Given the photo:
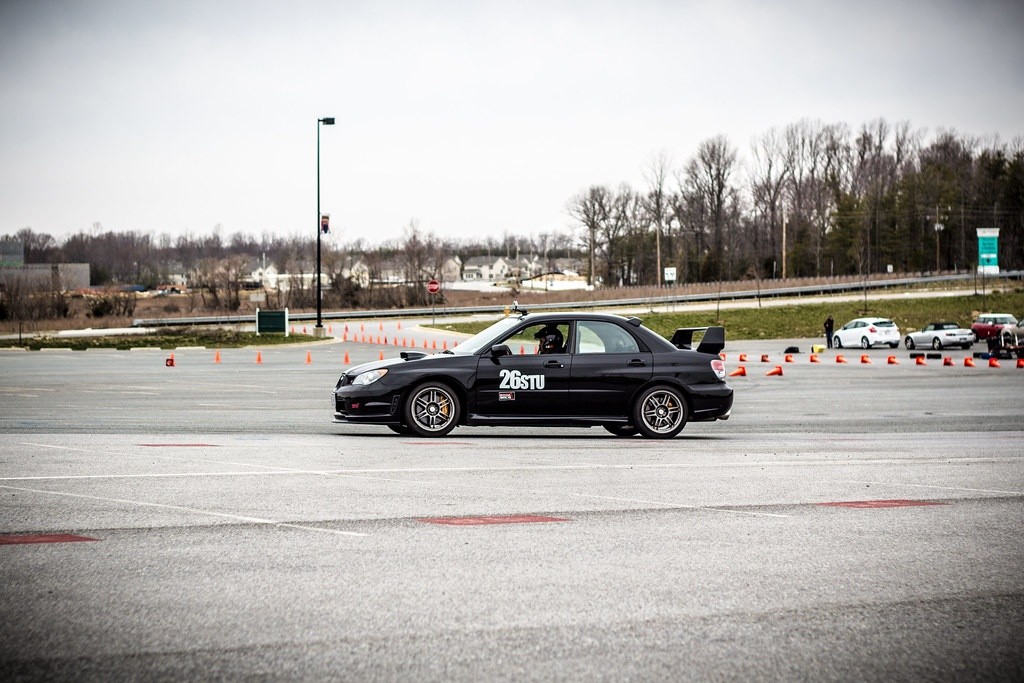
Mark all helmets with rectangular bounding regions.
[534,327,563,351]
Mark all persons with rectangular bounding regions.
[824,315,834,349]
[534,327,564,354]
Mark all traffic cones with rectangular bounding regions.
[944,357,954,365]
[534,344,539,355]
[785,355,794,363]
[520,346,525,355]
[888,356,899,364]
[767,366,782,376]
[256,351,263,364]
[344,351,350,365]
[729,366,747,377]
[762,355,770,362]
[740,354,748,362]
[170,353,175,366]
[916,356,927,366]
[861,354,872,363]
[964,357,976,367]
[305,350,312,364]
[291,321,458,351]
[214,350,222,363]
[988,358,1001,368]
[811,354,820,363]
[835,354,847,364]
[379,351,383,360]
[1016,357,1024,368]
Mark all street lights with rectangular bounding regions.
[312,117,335,337]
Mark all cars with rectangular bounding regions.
[831,316,900,350]
[1001,320,1024,353]
[332,306,733,439]
[971,313,1019,343]
[904,322,976,350]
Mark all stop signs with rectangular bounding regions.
[427,280,439,293]
